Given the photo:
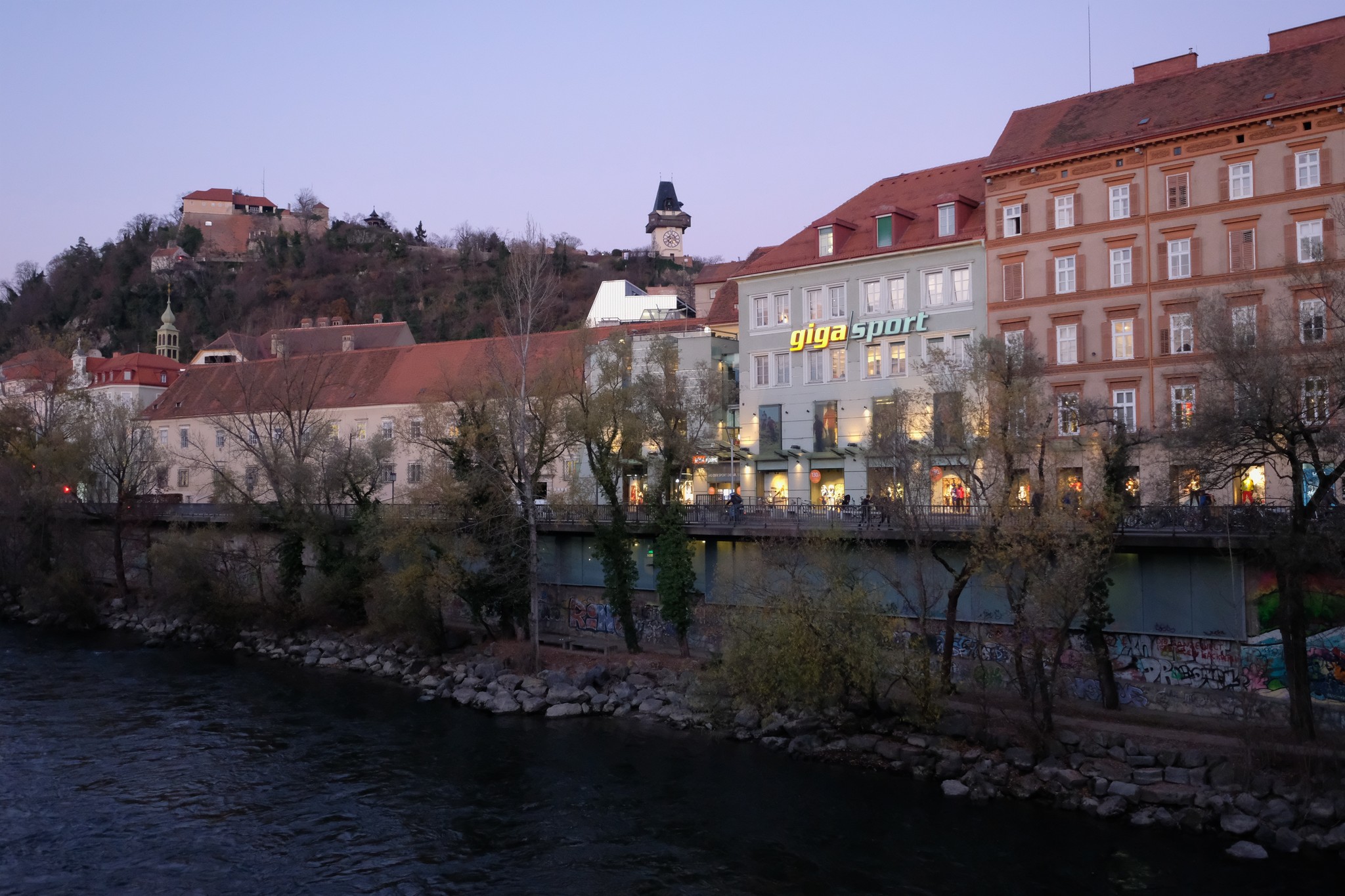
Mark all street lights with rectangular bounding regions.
[247,477,253,506]
[389,473,397,506]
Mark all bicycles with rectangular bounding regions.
[720,505,747,524]
[827,495,868,520]
[1122,498,1333,536]
[797,498,816,517]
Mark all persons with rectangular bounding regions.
[1060,491,1071,510]
[1240,472,1255,504]
[631,485,635,502]
[1018,486,1027,506]
[1068,476,1083,491]
[724,493,740,520]
[636,490,644,505]
[813,401,836,452]
[760,410,778,439]
[687,482,692,493]
[766,493,773,505]
[372,497,382,504]
[895,482,903,504]
[1197,490,1213,529]
[937,394,955,446]
[774,485,784,498]
[820,485,836,511]
[1189,476,1200,507]
[1030,488,1041,519]
[950,484,965,514]
[857,492,894,530]
[1317,487,1345,507]
